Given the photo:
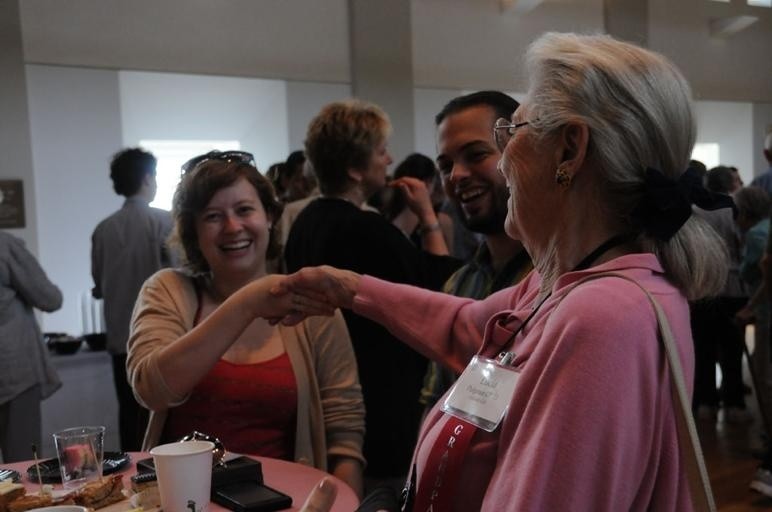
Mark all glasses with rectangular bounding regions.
[177,429,230,470]
[180,150,258,181]
[492,116,546,154]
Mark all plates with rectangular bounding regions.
[23,490,66,501]
[95,499,161,512]
[27,452,132,482]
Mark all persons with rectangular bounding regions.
[87,147,184,452]
[260,30,696,512]
[279,101,454,511]
[414,90,533,432]
[124,149,367,501]
[298,476,339,511]
[1,228,67,464]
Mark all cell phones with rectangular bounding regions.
[216,482,293,512]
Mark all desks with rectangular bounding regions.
[0,447,359,512]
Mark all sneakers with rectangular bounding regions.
[748,466,772,499]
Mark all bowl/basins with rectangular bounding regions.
[44,332,106,355]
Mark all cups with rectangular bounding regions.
[149,440,215,511]
[24,505,89,512]
[52,425,106,491]
